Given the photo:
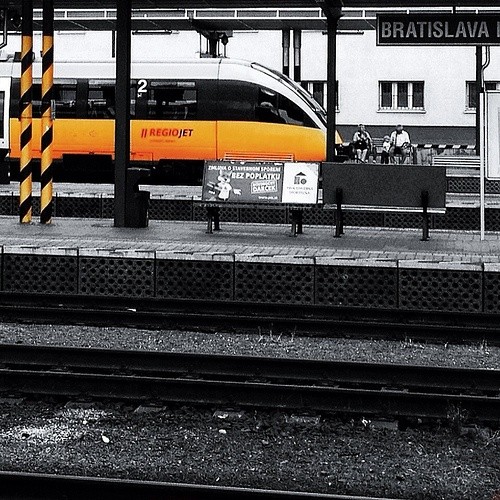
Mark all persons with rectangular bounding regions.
[353,124,373,164]
[389,125,411,164]
[380,134,391,164]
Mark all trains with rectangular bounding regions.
[0,58,344,162]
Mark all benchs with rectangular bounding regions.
[360,138,417,164]
[432,157,485,175]
[201,153,319,238]
[321,162,448,242]
